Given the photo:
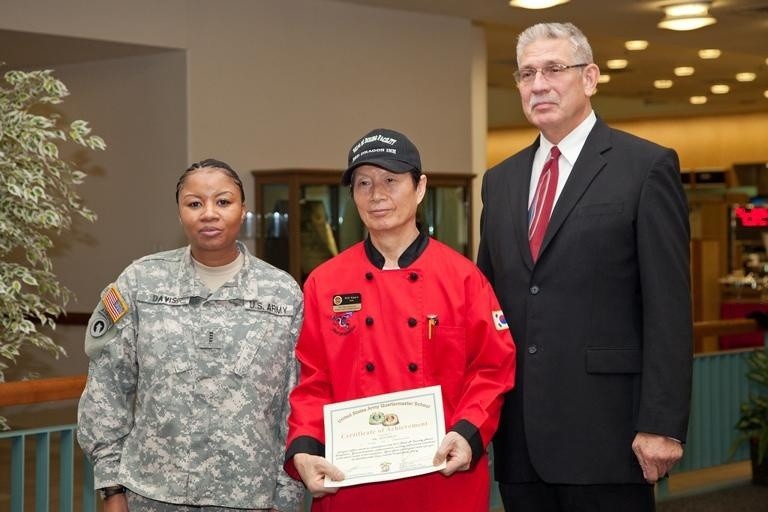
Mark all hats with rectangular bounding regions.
[341,128,421,185]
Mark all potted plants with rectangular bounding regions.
[723,347,768,493]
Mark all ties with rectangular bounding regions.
[527,147,561,264]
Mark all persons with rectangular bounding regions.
[475,22,693,512]
[77,160,304,512]
[282,130,518,512]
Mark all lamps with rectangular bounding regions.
[653,2,718,34]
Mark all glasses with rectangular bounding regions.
[512,63,587,85]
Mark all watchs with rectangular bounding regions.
[98,486,126,501]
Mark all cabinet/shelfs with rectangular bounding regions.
[246,162,478,308]
[678,157,768,358]
